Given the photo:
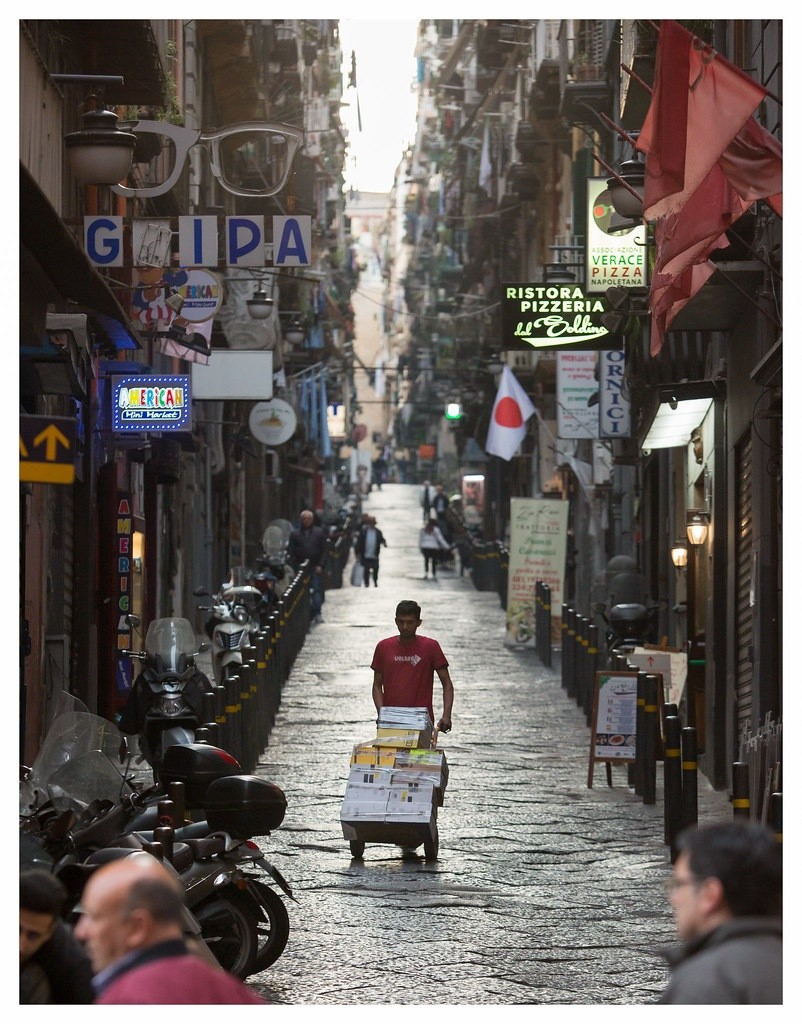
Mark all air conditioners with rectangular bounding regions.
[266,449,279,477]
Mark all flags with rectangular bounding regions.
[485,366,537,461]
[638,19,783,354]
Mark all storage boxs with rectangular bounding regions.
[162,744,243,807]
[339,706,449,823]
[204,775,287,833]
[611,603,648,637]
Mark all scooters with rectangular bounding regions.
[594,596,669,669]
[193,520,299,685]
[19,689,302,981]
[119,613,215,785]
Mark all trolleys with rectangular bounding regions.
[350,727,441,860]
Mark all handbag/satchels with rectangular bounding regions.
[351,561,366,586]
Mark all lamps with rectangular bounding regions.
[687,509,712,545]
[671,537,697,574]
[138,330,211,357]
[194,418,257,458]
[48,72,138,187]
[486,354,507,375]
[543,261,585,282]
[221,278,273,318]
[278,311,305,344]
[599,309,650,333]
[606,285,649,307]
[111,282,185,315]
[607,147,645,222]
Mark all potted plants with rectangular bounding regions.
[575,52,602,80]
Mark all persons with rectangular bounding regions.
[450,535,473,577]
[287,510,329,623]
[20,868,98,1005]
[354,465,371,495]
[432,484,449,520]
[419,479,437,523]
[351,514,387,587]
[370,599,455,734]
[371,457,386,491]
[75,847,273,1005]
[649,820,784,1006]
[420,519,451,580]
